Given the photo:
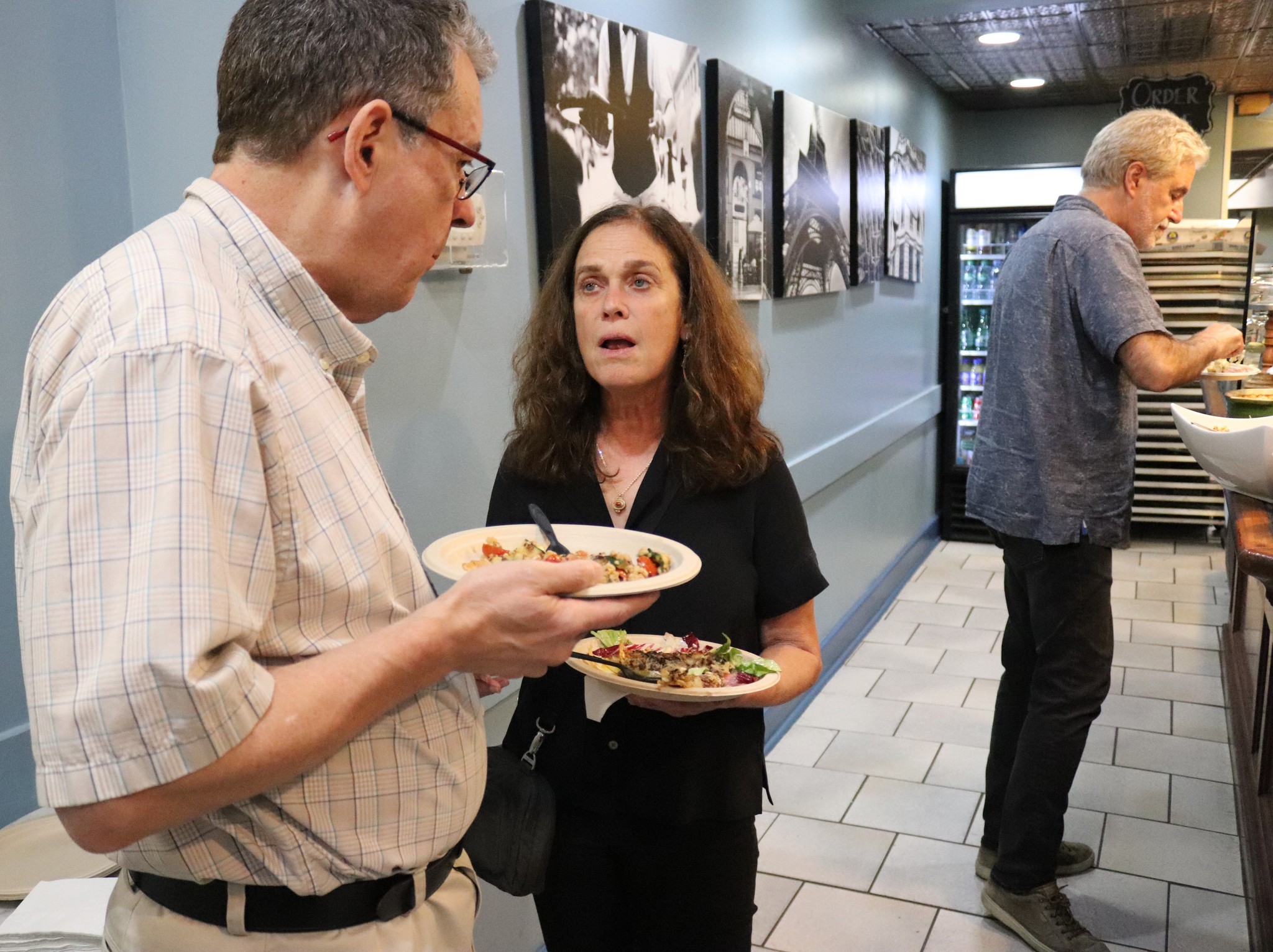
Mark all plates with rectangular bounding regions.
[1225,388,1273,405]
[1199,361,1262,381]
[564,634,780,702]
[421,523,702,599]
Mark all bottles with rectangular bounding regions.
[959,358,986,386]
[960,429,976,464]
[963,261,1003,300]
[965,222,1026,255]
[961,308,989,350]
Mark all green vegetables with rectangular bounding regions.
[605,548,664,573]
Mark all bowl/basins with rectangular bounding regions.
[1170,403,1273,503]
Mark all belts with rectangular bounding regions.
[122,834,469,934]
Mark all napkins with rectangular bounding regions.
[0,877,120,952]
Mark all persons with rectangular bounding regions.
[966,107,1247,952]
[10,1,662,952]
[485,204,830,952]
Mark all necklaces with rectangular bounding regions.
[595,443,653,514]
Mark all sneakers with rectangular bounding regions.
[980,877,1111,952]
[975,839,1095,880]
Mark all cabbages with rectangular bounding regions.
[590,628,783,677]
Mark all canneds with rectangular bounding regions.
[961,396,983,420]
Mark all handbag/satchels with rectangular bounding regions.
[460,738,555,897]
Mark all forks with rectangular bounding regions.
[570,652,662,683]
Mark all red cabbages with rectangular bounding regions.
[592,631,758,684]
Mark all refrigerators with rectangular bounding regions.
[934,161,1084,544]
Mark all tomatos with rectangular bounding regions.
[481,543,660,578]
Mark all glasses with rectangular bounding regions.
[328,105,496,200]
